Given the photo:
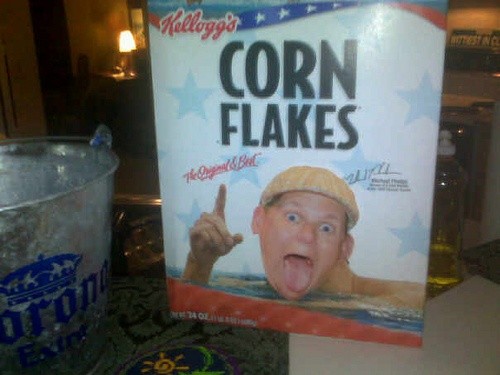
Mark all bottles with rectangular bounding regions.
[426,129,465,298]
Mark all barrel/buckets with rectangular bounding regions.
[0,124,120,375]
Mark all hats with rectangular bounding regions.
[259,165,359,230]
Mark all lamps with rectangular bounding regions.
[114,29,137,78]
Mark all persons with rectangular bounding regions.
[180,165,425,319]
